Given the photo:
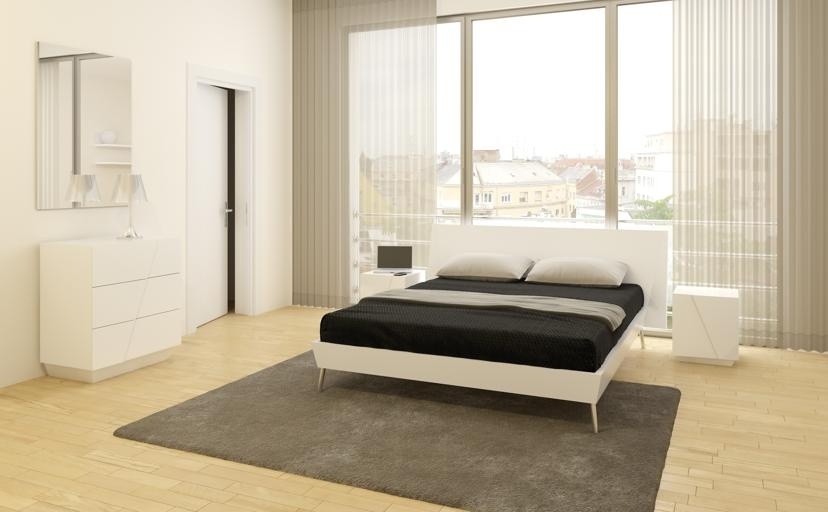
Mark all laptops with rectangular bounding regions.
[373,245,412,273]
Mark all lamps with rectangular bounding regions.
[110,174,149,239]
[69,174,104,208]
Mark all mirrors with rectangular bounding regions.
[37,39,133,210]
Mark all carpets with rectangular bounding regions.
[113,349,681,512]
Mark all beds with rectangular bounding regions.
[310,223,668,434]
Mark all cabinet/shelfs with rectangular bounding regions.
[39,233,182,383]
[94,145,133,165]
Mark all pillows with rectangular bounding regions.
[524,256,630,289]
[435,253,535,283]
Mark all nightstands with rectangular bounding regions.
[671,285,739,367]
[359,269,425,301]
